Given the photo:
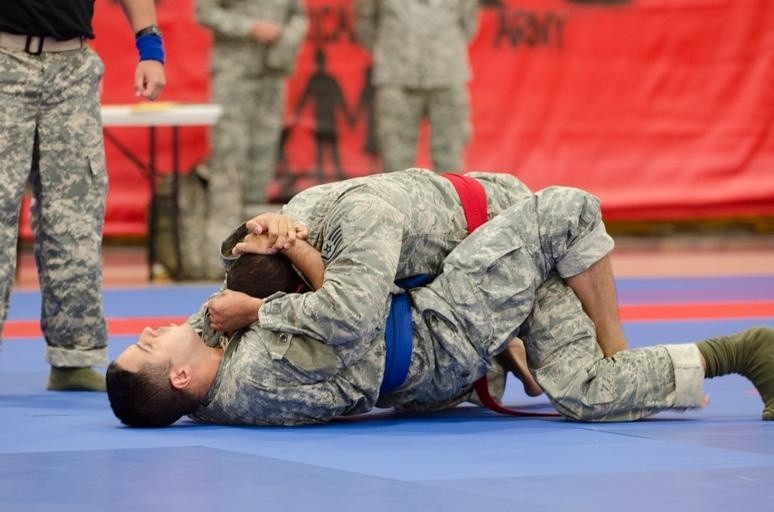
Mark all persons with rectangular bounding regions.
[206,167,774,422]
[353,0,481,174]
[0,0,167,392]
[195,0,312,283]
[106,185,632,429]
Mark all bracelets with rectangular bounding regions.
[135,35,164,64]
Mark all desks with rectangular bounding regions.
[102,102,224,282]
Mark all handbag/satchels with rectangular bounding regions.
[153,164,244,282]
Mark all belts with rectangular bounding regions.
[0,32,87,55]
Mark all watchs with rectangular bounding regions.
[135,24,162,38]
